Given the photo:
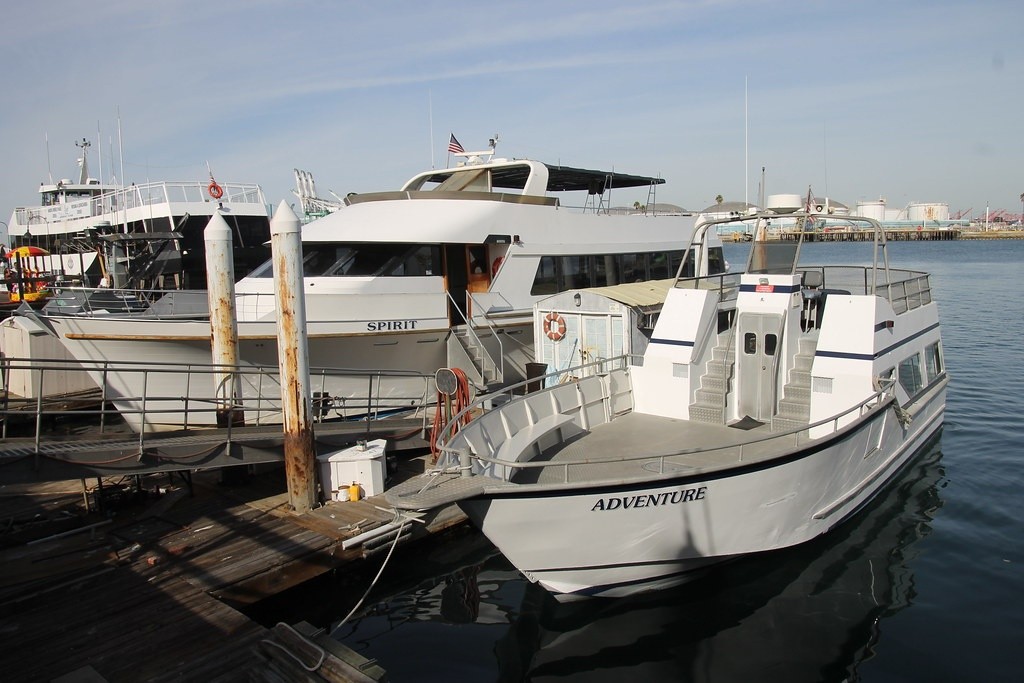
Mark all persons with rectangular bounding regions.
[0,244,18,302]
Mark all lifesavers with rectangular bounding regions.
[824,227,829,233]
[208,184,223,198]
[543,313,566,340]
[492,256,504,276]
[917,226,922,230]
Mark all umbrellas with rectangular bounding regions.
[5,246,50,258]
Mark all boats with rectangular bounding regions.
[344,164,952,602]
[0,135,732,434]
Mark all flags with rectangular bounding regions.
[449,134,468,157]
